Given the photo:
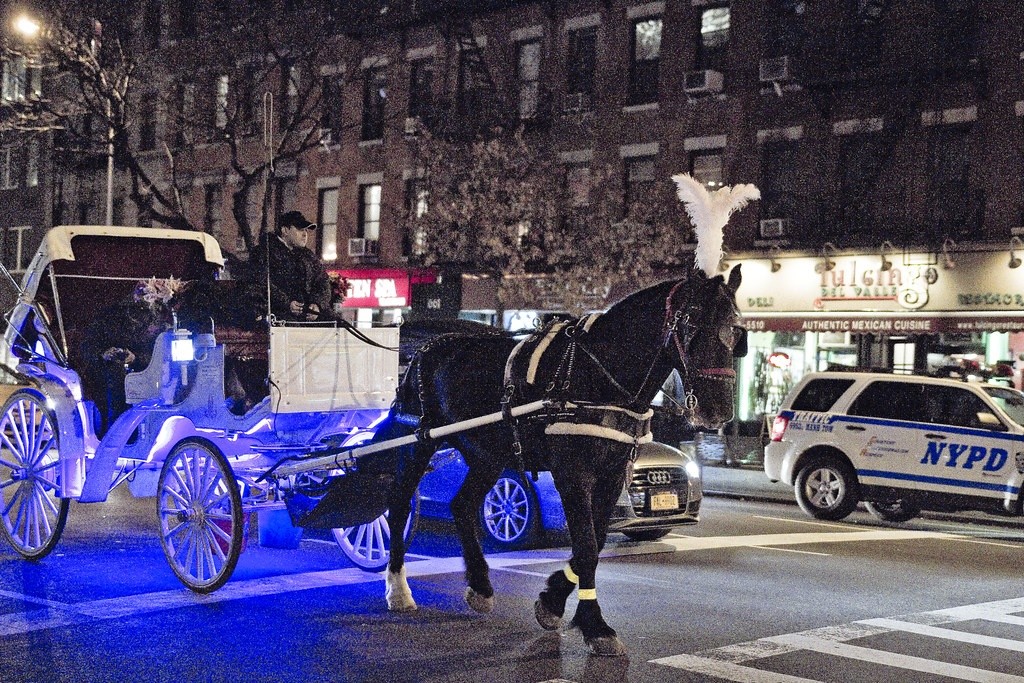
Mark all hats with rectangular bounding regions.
[278,211,316,231]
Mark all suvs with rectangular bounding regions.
[764,364,1024,523]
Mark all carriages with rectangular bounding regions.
[0,225,748,655]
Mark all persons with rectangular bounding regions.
[649,368,685,449]
[74,291,153,421]
[237,211,333,402]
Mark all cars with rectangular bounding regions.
[0,334,58,448]
[419,441,703,550]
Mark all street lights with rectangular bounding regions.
[16,19,113,226]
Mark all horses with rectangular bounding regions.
[384,263,742,656]
